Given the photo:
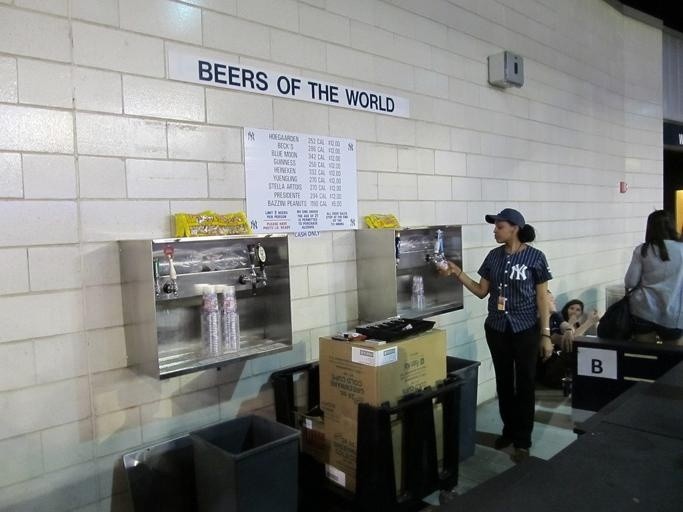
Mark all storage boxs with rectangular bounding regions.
[318,327,447,493]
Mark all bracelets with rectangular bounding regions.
[541,326,552,331]
[541,332,554,339]
[458,269,463,278]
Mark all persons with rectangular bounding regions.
[597,211,683,339]
[437,208,555,459]
[537,287,606,385]
[560,299,584,329]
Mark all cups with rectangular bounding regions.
[433,253,449,271]
[201,286,241,357]
[410,275,425,311]
[560,377,573,397]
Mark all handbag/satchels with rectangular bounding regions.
[597,296,628,341]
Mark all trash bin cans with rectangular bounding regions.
[447,356,481,456]
[189,413,302,512]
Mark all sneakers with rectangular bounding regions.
[492,430,529,462]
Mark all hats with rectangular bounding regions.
[485,209,525,230]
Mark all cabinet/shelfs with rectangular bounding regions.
[354,224,464,324]
[118,229,295,379]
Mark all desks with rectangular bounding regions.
[428,361,683,512]
